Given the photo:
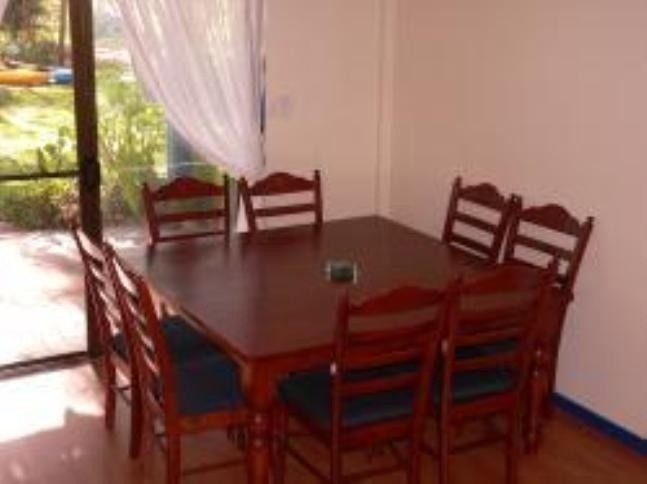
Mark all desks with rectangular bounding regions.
[113,212,547,483]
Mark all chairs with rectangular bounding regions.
[495,193,593,424]
[99,234,245,484]
[235,166,328,239]
[139,174,241,243]
[434,173,518,269]
[70,226,219,461]
[273,277,461,482]
[418,257,560,482]
